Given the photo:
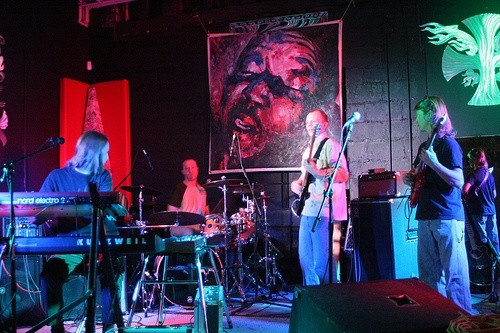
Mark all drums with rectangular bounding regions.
[199,213,236,248]
[229,211,258,245]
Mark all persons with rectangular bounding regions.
[168,158,210,230]
[36,131,113,326]
[403,95,472,312]
[463,147,500,257]
[290,109,349,286]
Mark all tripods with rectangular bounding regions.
[216,182,289,305]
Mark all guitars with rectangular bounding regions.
[294,122,322,217]
[409,115,447,209]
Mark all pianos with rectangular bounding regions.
[0,189,155,333]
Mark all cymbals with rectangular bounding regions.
[228,182,265,194]
[201,178,246,189]
[120,184,165,194]
[148,209,207,227]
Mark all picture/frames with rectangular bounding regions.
[208,19,348,174]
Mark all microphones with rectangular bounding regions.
[48,136,64,144]
[342,111,362,128]
[231,133,236,157]
[142,146,156,170]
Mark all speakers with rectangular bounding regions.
[287,277,473,333]
[0,254,44,326]
[351,197,421,284]
